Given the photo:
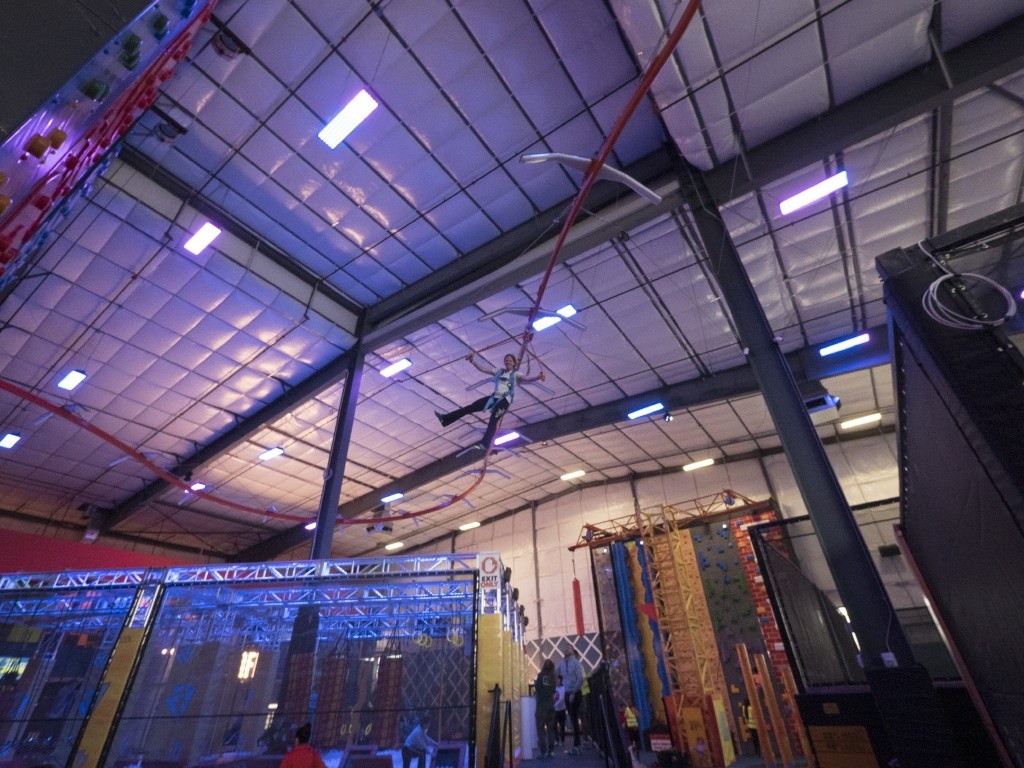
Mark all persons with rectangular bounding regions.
[554,675,567,746]
[559,643,583,756]
[535,659,557,758]
[434,353,545,450]
[403,716,438,768]
[280,721,327,768]
[624,702,640,745]
[618,702,627,723]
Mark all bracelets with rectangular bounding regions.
[571,692,575,695]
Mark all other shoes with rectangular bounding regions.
[560,741,565,748]
[434,410,444,425]
[474,444,487,451]
[564,746,579,755]
[548,750,555,756]
[555,739,559,745]
[535,754,546,759]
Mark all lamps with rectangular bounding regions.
[664,411,674,422]
[58,334,105,391]
[184,188,230,256]
[318,31,396,151]
